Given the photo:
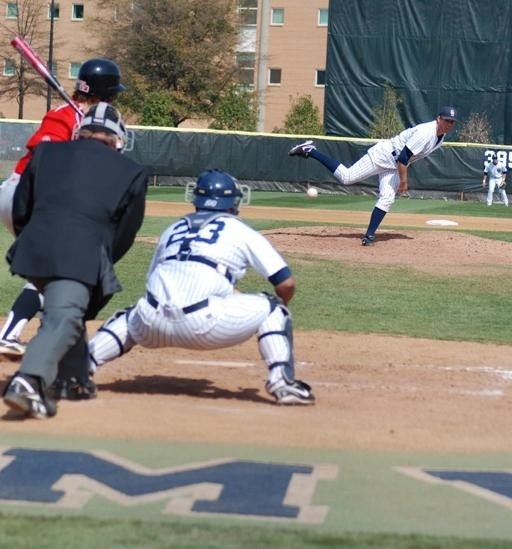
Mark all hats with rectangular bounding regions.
[439,106,457,123]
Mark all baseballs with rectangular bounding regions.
[308,188,318,198]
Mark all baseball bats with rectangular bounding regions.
[11,35,84,119]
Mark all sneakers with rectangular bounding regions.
[362,234,375,246]
[287,140,315,159]
[4,374,47,418]
[0,339,26,356]
[56,378,97,400]
[274,384,315,405]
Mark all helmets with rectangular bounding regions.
[71,102,135,155]
[185,171,250,210]
[77,59,125,95]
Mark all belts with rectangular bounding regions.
[147,292,207,314]
[392,150,399,163]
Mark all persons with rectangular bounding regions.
[482,155,509,207]
[288,106,459,248]
[0,58,127,358]
[2,102,148,421]
[47,169,316,407]
